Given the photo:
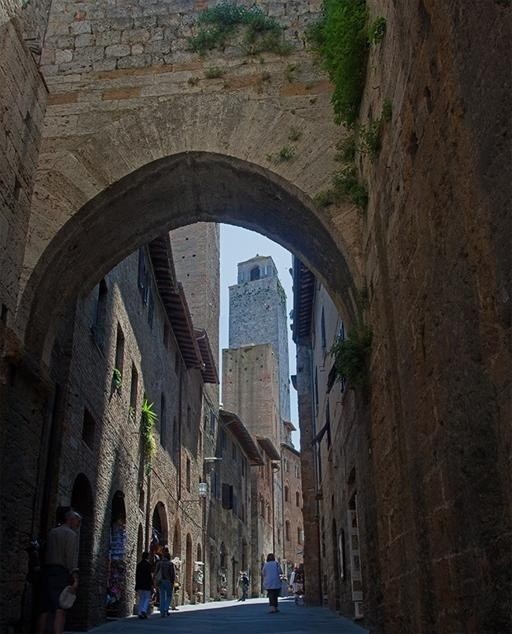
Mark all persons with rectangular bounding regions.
[262,553,283,613]
[135,552,176,619]
[39,510,82,634]
[289,562,305,607]
[236,571,250,601]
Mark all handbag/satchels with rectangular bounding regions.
[154,561,162,587]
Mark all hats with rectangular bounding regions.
[58,585,77,609]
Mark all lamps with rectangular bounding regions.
[198,481,208,498]
